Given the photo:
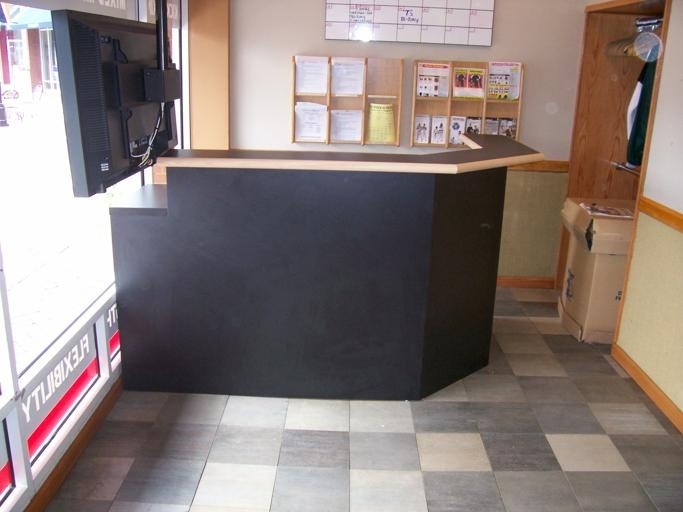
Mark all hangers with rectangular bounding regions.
[603,23,659,58]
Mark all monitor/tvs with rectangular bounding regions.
[51,10,178,197]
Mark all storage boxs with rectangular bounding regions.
[557,196,635,344]
